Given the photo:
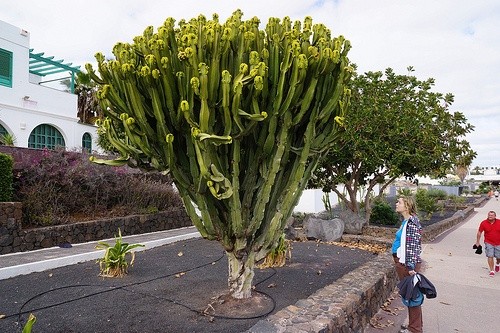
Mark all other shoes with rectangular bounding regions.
[495,262,500,272]
[400,323,423,333]
[489,271,494,277]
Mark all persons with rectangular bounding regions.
[494,191,499,200]
[476,211,500,276]
[391,196,423,333]
